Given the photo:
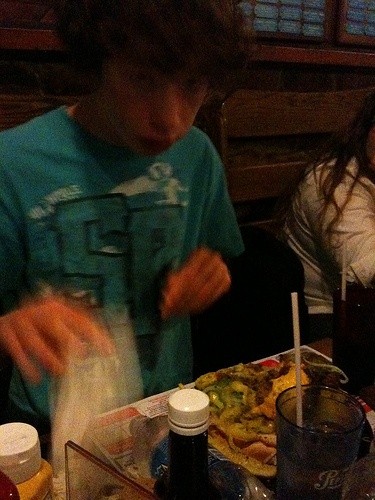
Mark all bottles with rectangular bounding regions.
[129,414,272,500]
[0,422,54,500]
[158,388,220,500]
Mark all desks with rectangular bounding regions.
[39,337,375,500]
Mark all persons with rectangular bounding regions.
[268,91,375,337]
[1,2,254,448]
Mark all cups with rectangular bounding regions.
[275,384,365,500]
[332,285,375,394]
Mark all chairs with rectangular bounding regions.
[213,89,375,359]
